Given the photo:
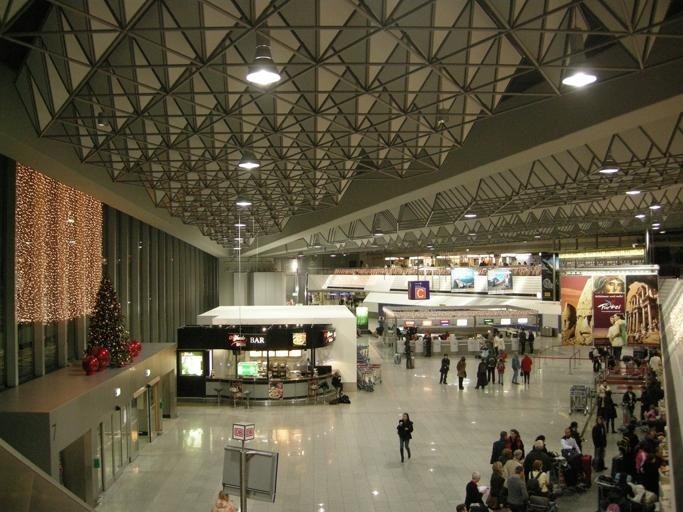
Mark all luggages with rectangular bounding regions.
[581,455,592,483]
[611,456,623,478]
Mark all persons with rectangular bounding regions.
[211,490,238,512]
[593,276,625,293]
[608,316,623,361]
[397,413,413,463]
[339,292,354,308]
[612,313,628,346]
[423,331,432,357]
[438,332,449,340]
[456,329,669,512]
[374,323,418,369]
[332,369,343,395]
[439,353,451,384]
[384,257,537,276]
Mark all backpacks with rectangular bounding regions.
[527,471,543,496]
[618,436,631,453]
[605,497,625,512]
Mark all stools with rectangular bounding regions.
[243,390,252,409]
[310,388,319,402]
[321,385,328,400]
[214,388,223,408]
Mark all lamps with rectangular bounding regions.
[561,26,599,88]
[296,226,541,259]
[233,195,253,250]
[238,151,261,168]
[464,206,477,218]
[599,150,620,174]
[625,177,666,235]
[246,21,281,86]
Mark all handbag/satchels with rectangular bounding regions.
[592,457,599,471]
[486,495,499,510]
[564,464,581,487]
[562,449,573,457]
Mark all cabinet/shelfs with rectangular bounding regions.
[271,359,286,379]
[568,383,591,415]
